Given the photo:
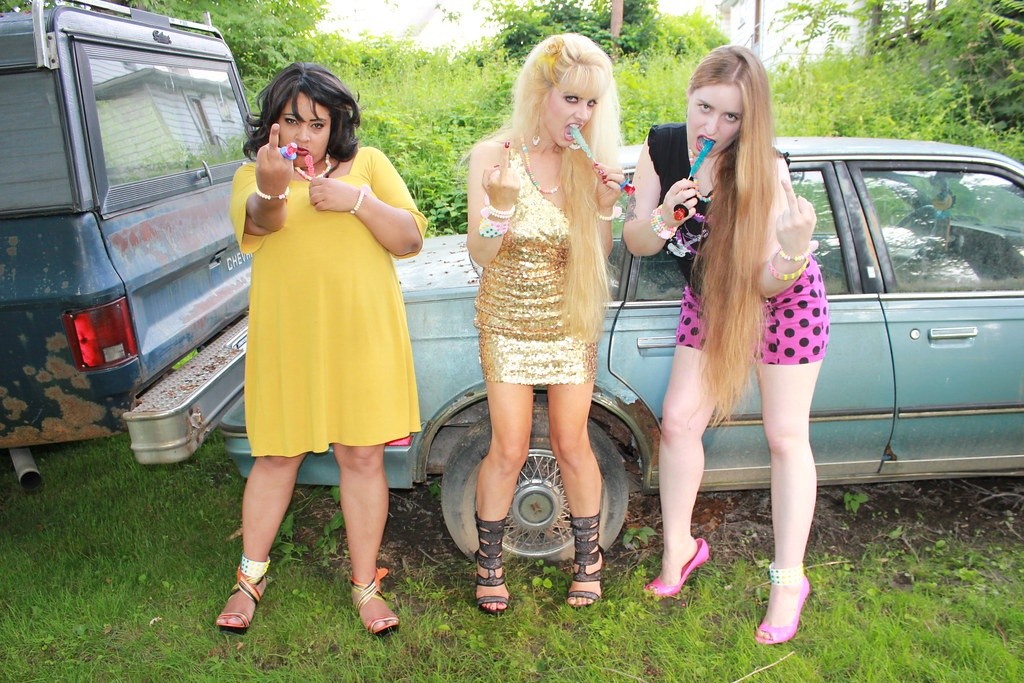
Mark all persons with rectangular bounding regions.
[626,47,828,643]
[216,61,429,638]
[460,33,625,611]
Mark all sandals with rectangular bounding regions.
[350,575,401,635]
[215,565,269,633]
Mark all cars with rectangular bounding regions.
[217,135,1024,565]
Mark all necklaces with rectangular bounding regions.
[689,149,715,202]
[518,133,562,194]
[295,154,332,182]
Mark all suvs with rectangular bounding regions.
[0,0,252,493]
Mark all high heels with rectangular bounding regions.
[471,512,510,613]
[566,512,604,609]
[645,538,709,599]
[753,576,809,644]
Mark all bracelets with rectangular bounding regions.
[477,205,516,237]
[255,184,290,199]
[599,207,622,222]
[351,185,370,214]
[650,205,676,240]
[766,244,810,281]
